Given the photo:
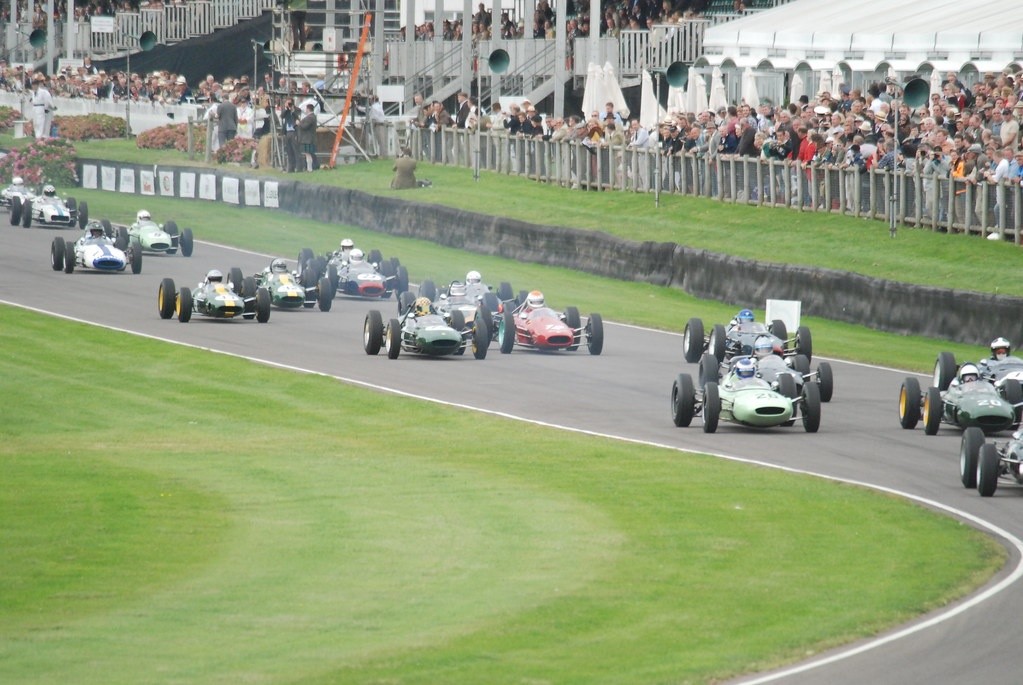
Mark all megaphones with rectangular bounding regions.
[888,78,930,107]
[21,28,46,48]
[480,49,510,74]
[129,30,157,52]
[651,60,688,88]
[258,40,269,50]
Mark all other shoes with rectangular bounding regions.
[987,233,998,240]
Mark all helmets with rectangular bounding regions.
[12,177,24,188]
[349,249,363,264]
[736,310,754,325]
[43,184,55,198]
[137,210,150,222]
[86,221,105,238]
[990,337,1011,350]
[527,291,545,310]
[465,271,481,287]
[270,258,287,274]
[733,359,756,380]
[206,269,223,284]
[753,335,774,358]
[340,239,354,252]
[959,362,980,383]
[415,297,431,316]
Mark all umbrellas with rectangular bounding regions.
[582,60,842,123]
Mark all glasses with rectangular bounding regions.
[962,375,977,381]
[492,76,1021,182]
[993,348,1008,354]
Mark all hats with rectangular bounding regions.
[814,106,826,115]
[1003,108,1011,115]
[875,111,888,121]
[604,113,616,120]
[859,121,872,131]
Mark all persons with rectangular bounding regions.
[13,178,55,199]
[193,72,324,173]
[401,0,746,71]
[415,271,545,316]
[627,67,1023,244]
[137,210,150,223]
[85,221,106,239]
[204,239,363,284]
[737,309,774,378]
[355,95,388,158]
[990,337,1011,361]
[957,362,980,384]
[0,57,192,141]
[0,0,186,35]
[276,0,307,51]
[410,91,625,191]
[391,148,416,190]
